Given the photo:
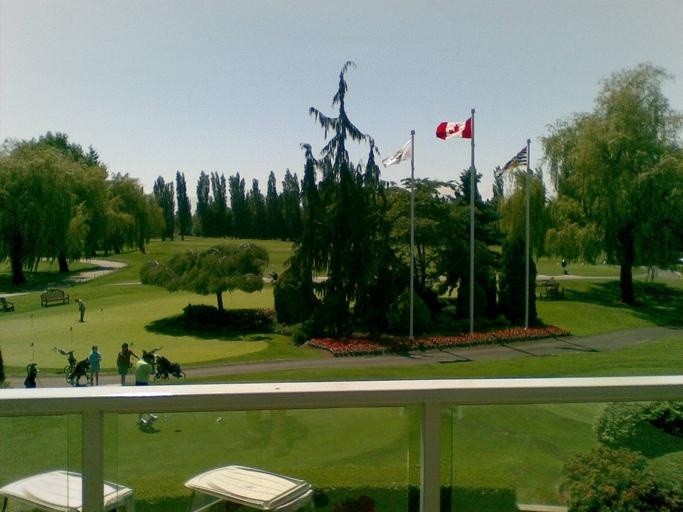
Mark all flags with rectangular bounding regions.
[496,146,527,178]
[381,135,412,168]
[436,117,471,143]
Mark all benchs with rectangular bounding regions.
[40,288,70,307]
[0,296,15,312]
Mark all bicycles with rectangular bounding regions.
[63,365,95,386]
[152,368,185,382]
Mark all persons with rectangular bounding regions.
[117,343,141,385]
[75,298,87,323]
[23,365,36,388]
[134,352,158,426]
[561,258,568,276]
[88,343,103,387]
[269,271,278,286]
